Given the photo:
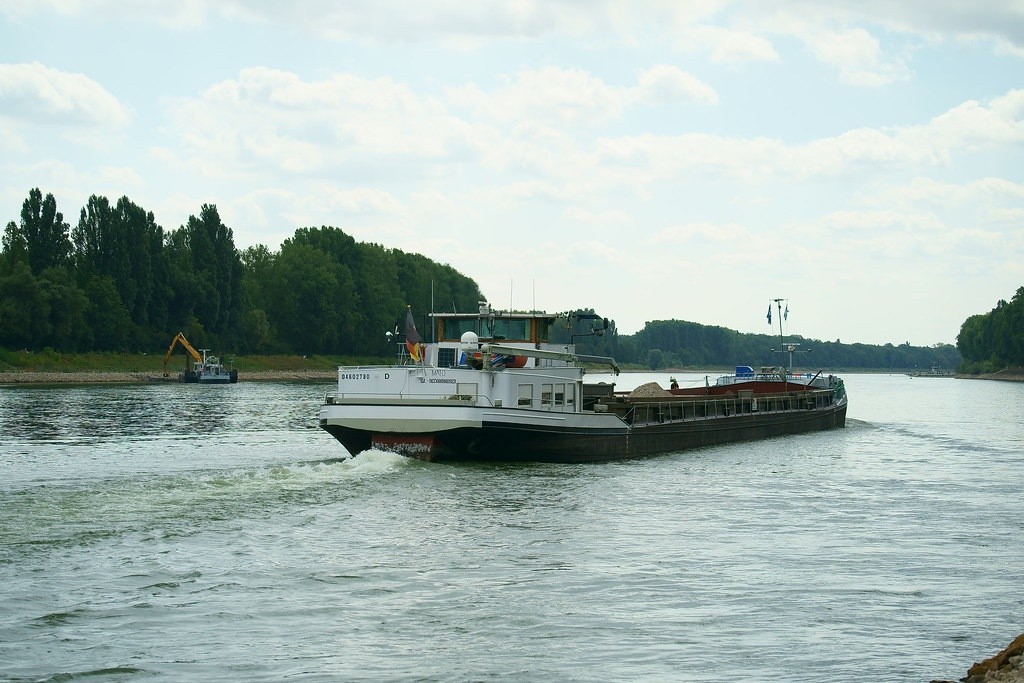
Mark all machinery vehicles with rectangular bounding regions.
[162,332,222,377]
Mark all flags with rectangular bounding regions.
[784,304,789,321]
[405,305,424,362]
[766,303,772,325]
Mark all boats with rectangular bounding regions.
[905,361,955,377]
[465,350,528,369]
[319,279,849,466]
